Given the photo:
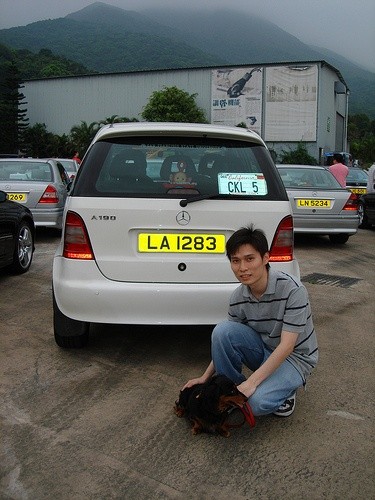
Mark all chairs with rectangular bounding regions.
[109,149,157,194]
[198,152,245,195]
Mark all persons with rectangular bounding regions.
[367,162,375,193]
[182,228,318,416]
[73,151,81,164]
[328,154,349,189]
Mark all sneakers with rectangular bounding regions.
[273,392,296,417]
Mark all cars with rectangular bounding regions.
[30,158,81,187]
[1,191,36,277]
[324,167,375,229]
[0,159,70,232]
[50,121,305,350]
[275,165,360,245]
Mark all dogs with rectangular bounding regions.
[172,374,249,438]
